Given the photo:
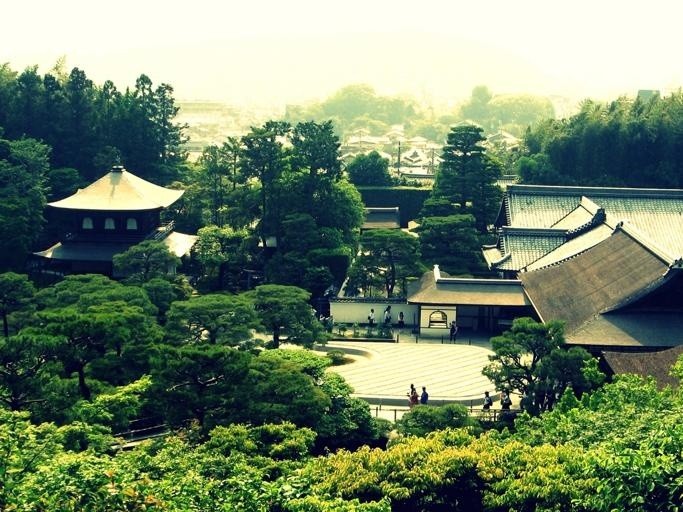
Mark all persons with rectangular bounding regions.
[384,305,392,324]
[319,313,325,321]
[484,392,491,409]
[369,308,376,327]
[407,384,428,408]
[449,321,458,343]
[398,311,405,328]
[502,389,511,409]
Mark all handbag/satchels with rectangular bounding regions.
[504,400,512,407]
